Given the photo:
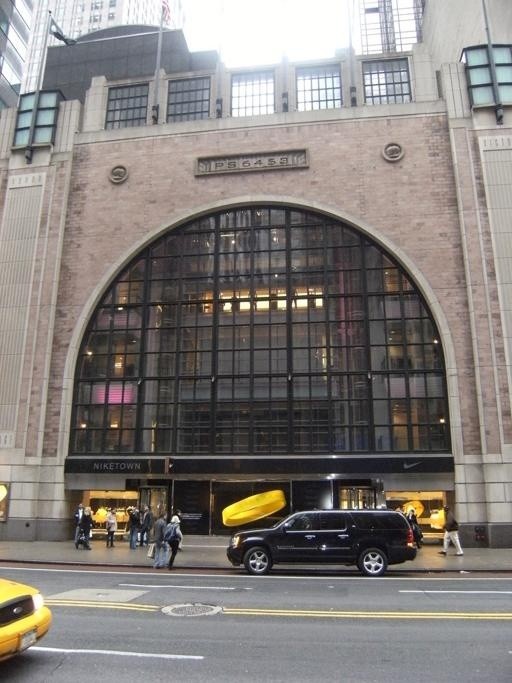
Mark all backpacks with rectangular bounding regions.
[162,522,179,545]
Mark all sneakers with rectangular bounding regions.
[438,551,464,556]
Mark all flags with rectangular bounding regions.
[162,0,172,25]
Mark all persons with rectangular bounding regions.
[74,504,184,570]
[437,503,464,556]
[407,509,420,549]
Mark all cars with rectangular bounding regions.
[0,577,52,663]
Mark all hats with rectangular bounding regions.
[443,505,449,509]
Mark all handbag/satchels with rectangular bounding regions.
[106,522,112,530]
[147,543,155,559]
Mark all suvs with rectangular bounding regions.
[227,508,418,577]
